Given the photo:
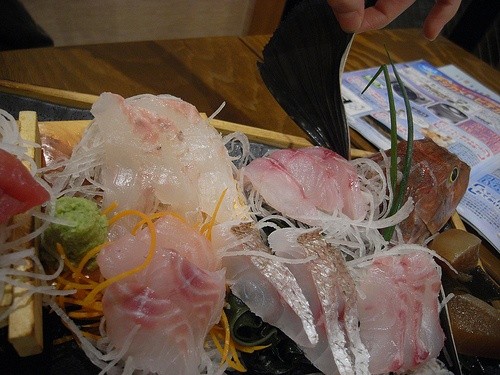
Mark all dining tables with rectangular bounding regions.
[0,29,500,375]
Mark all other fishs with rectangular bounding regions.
[372,138,471,248]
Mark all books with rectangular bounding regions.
[341,58,500,253]
